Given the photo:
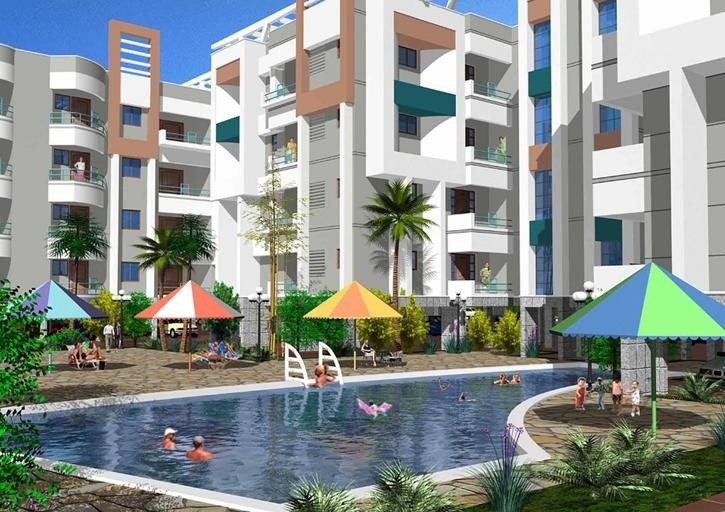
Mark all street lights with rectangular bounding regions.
[111,289,132,349]
[572,279,607,386]
[449,291,467,353]
[248,286,269,350]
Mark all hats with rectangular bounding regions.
[164,427,178,436]
[193,436,204,444]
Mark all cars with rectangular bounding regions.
[466,307,477,320]
[163,320,200,339]
